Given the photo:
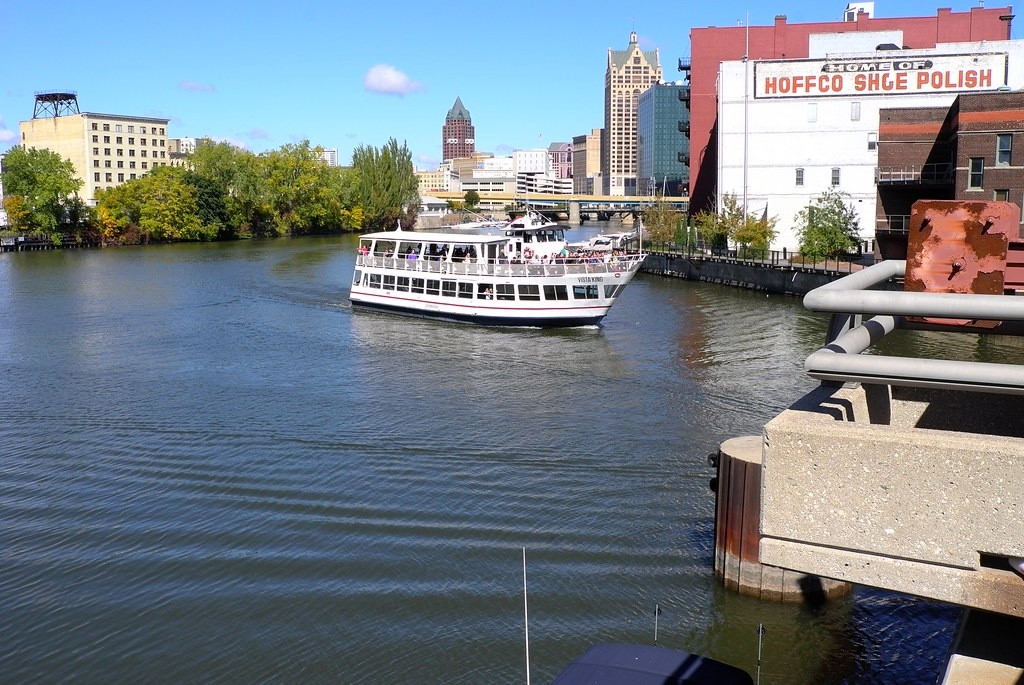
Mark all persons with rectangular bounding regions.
[483,287,501,298]
[497,244,629,275]
[404,243,476,258]
[355,239,476,275]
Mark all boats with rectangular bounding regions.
[579,214,590,221]
[597,213,610,220]
[348,202,648,328]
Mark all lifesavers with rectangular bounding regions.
[524,247,533,259]
